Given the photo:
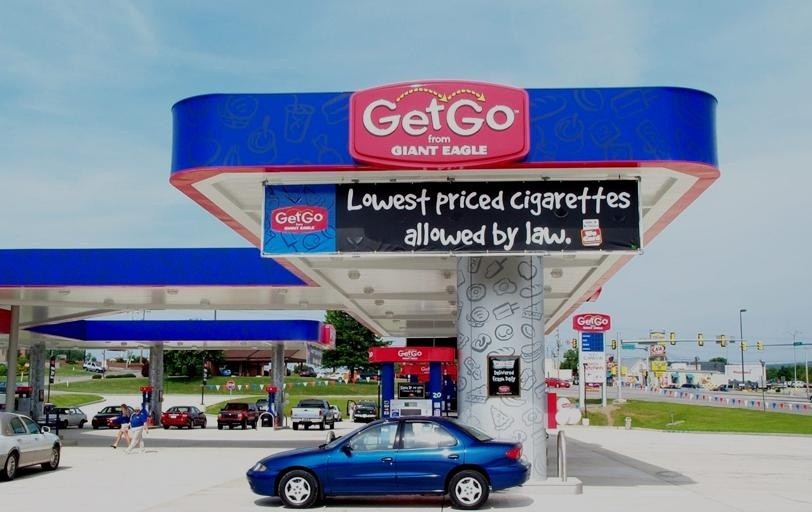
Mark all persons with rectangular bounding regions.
[123,406,149,454]
[110,404,131,447]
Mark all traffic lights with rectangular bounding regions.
[612,340,616,349]
[757,341,762,350]
[573,339,577,348]
[742,342,746,351]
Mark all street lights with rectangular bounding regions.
[739,308,748,383]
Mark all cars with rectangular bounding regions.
[544,377,601,389]
[217,368,380,383]
[662,379,812,402]
[0,410,63,483]
[0,381,25,394]
[82,361,106,373]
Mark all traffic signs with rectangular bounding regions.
[622,343,635,349]
[793,342,802,346]
